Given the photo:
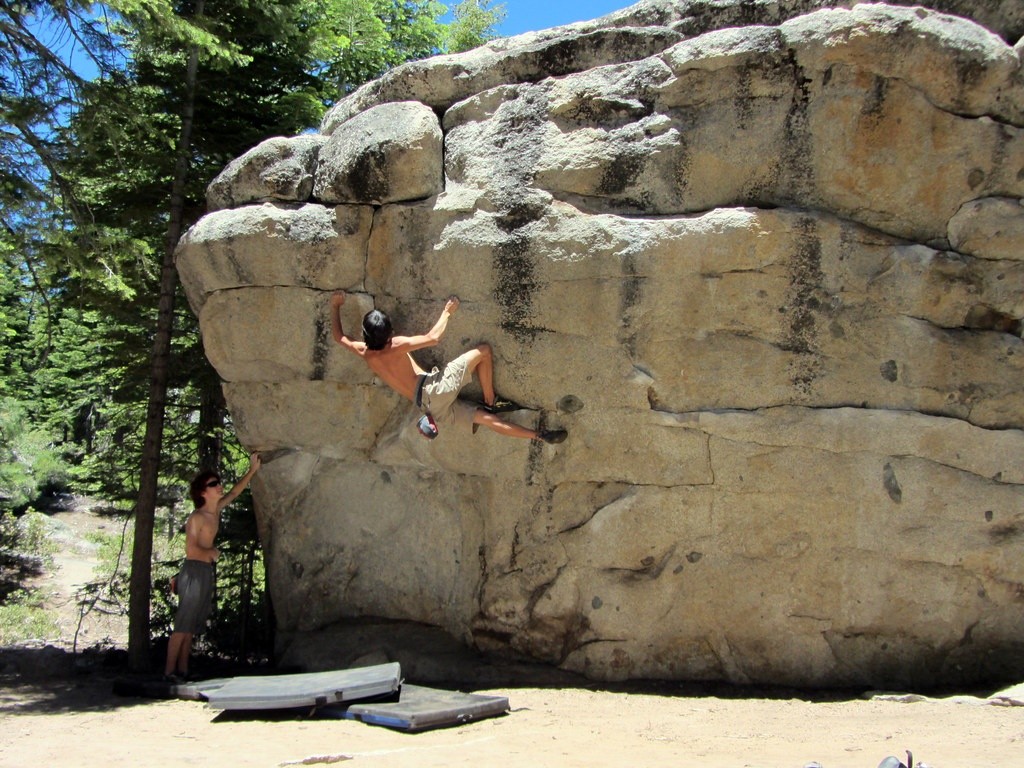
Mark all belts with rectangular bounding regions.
[416,373,429,407]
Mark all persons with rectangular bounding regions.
[157,450,261,699]
[331,290,568,445]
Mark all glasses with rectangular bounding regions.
[206,480,221,487]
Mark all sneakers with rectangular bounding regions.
[534,429,568,444]
[483,395,519,413]
